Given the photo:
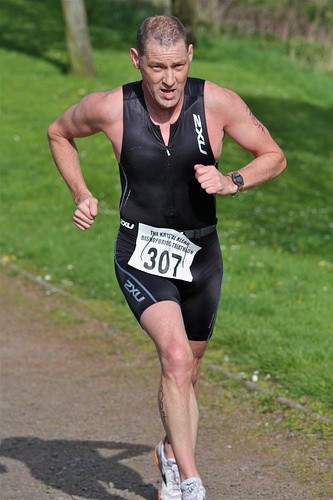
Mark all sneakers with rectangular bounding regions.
[155,433,182,500]
[180,477,205,500]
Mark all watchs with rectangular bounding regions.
[226,170,245,196]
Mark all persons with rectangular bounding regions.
[47,13,289,500]
